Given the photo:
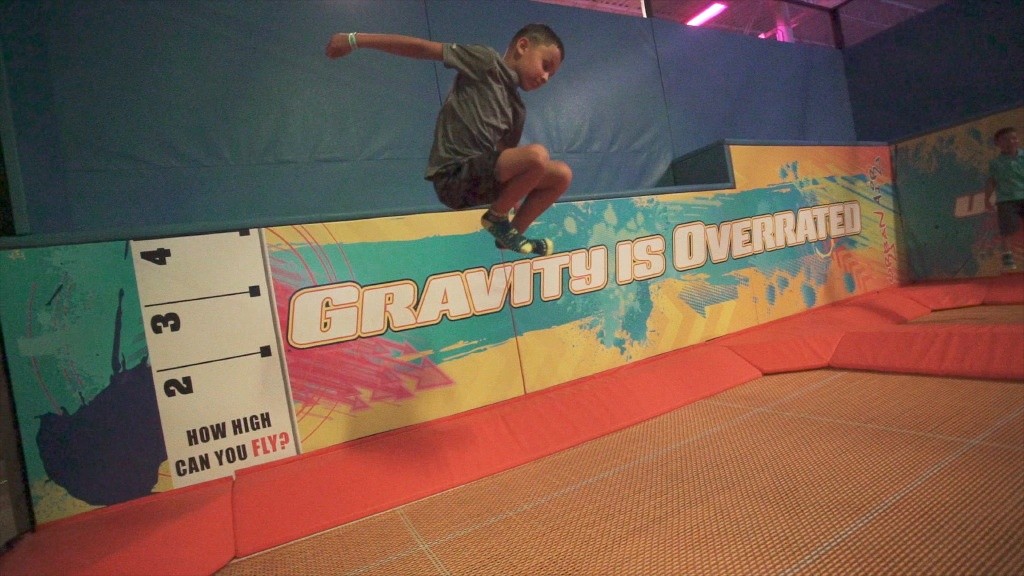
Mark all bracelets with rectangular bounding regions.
[347,31,359,52]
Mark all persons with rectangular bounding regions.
[983,126,1024,270]
[324,22,573,256]
